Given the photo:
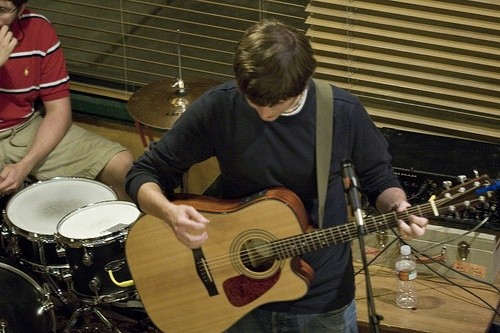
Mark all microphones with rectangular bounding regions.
[341,157,364,235]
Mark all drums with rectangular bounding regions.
[2,175,119,275]
[55,199,146,305]
[0,263,57,333]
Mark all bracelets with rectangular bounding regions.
[392,203,399,210]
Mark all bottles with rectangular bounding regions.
[394,245,417,309]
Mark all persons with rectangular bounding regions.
[0,0,132,201]
[125,21,429,333]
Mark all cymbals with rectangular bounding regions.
[127,77,223,130]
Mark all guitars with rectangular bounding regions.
[125,169,497,333]
